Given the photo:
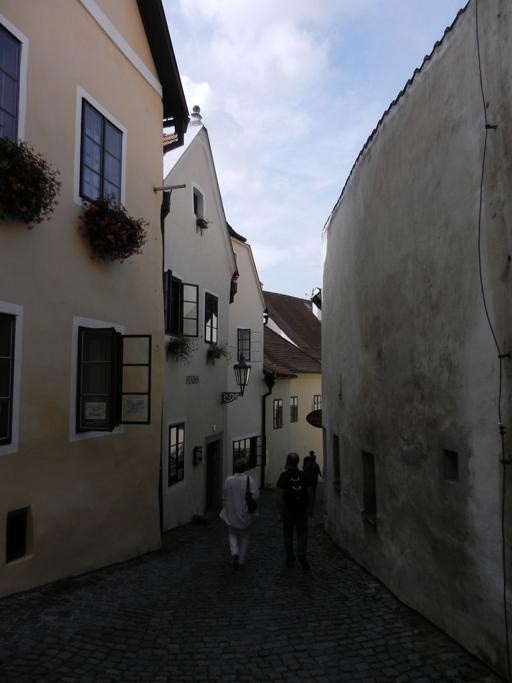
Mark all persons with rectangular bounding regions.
[306,455,321,493]
[217,456,259,571]
[275,452,315,567]
[302,450,314,471]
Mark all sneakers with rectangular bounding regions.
[285,553,310,571]
[232,553,248,568]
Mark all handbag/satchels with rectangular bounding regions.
[245,476,257,508]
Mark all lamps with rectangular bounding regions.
[222,354,251,404]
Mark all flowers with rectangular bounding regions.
[79,192,148,264]
[0,140,62,231]
